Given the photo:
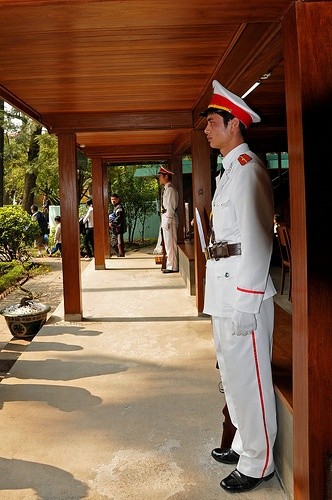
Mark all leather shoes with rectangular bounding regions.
[220,468,275,492]
[211,448,240,464]
[163,269,179,273]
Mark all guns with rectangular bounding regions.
[194,207,237,449]
[158,190,167,271]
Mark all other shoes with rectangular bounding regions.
[37,255,43,258]
[46,248,50,254]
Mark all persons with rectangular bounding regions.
[79,199,94,260]
[158,165,179,273]
[24,205,49,257]
[48,216,62,257]
[109,193,127,257]
[201,79,278,493]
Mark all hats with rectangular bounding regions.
[156,165,174,175]
[199,79,262,130]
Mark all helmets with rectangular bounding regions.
[109,214,115,221]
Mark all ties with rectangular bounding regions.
[220,164,225,178]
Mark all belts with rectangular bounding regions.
[207,242,242,261]
[162,208,177,214]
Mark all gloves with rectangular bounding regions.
[230,310,257,336]
